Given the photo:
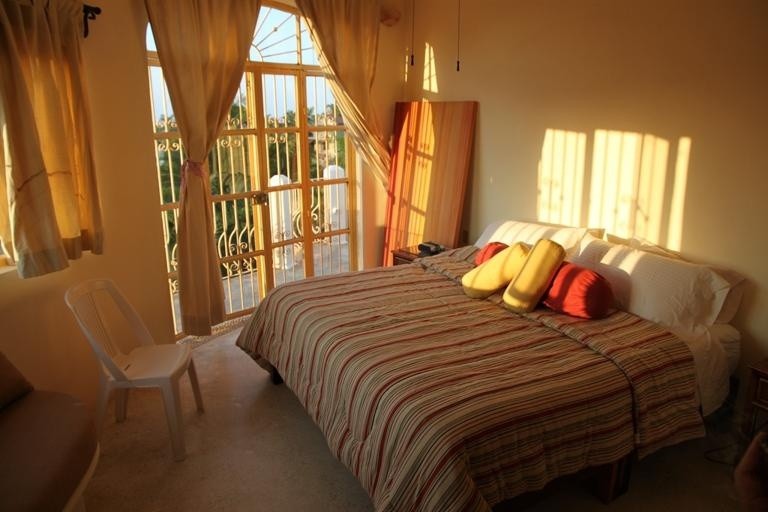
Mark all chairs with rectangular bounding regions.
[0,350,100,512]
[64,279,204,461]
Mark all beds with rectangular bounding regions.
[236,245,741,512]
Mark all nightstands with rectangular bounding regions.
[733,358,768,470]
[391,245,452,266]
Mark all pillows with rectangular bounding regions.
[461,220,745,341]
